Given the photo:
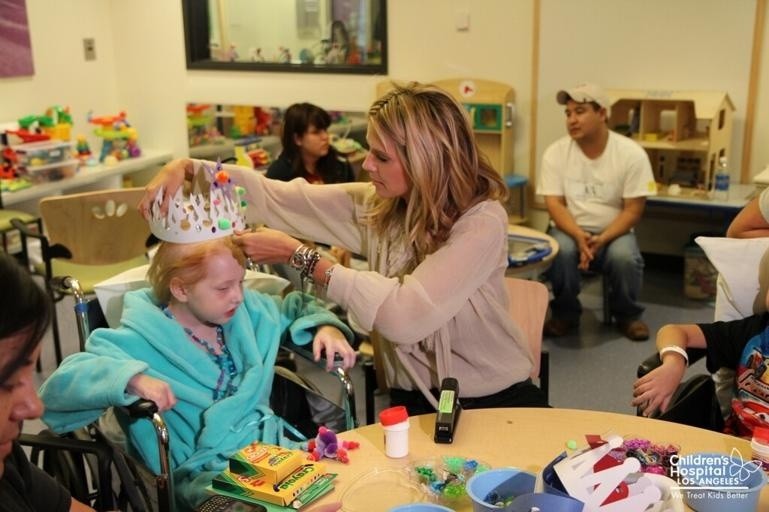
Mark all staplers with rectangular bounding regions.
[434,377,461,443]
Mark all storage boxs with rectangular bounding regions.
[3,122,82,185]
[189,103,259,147]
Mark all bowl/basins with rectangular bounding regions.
[678,452,769,512]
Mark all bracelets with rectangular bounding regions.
[300,250,322,280]
[322,263,336,288]
[659,345,690,368]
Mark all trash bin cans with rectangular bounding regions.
[683,240,718,300]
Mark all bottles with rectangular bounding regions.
[378,405,412,458]
[714,156,729,201]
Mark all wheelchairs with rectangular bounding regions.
[13,254,361,512]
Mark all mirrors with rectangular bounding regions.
[185,101,375,187]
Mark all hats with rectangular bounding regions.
[556,84,611,117]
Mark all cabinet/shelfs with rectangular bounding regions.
[371,78,530,227]
[587,86,737,201]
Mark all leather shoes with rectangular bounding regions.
[543,319,566,338]
[618,319,650,341]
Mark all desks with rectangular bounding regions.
[189,117,369,170]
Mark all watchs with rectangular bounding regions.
[287,244,313,271]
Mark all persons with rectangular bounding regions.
[0,250,100,512]
[630,283,769,452]
[0,100,361,194]
[727,181,768,241]
[135,82,553,418]
[39,211,357,511]
[535,84,660,341]
